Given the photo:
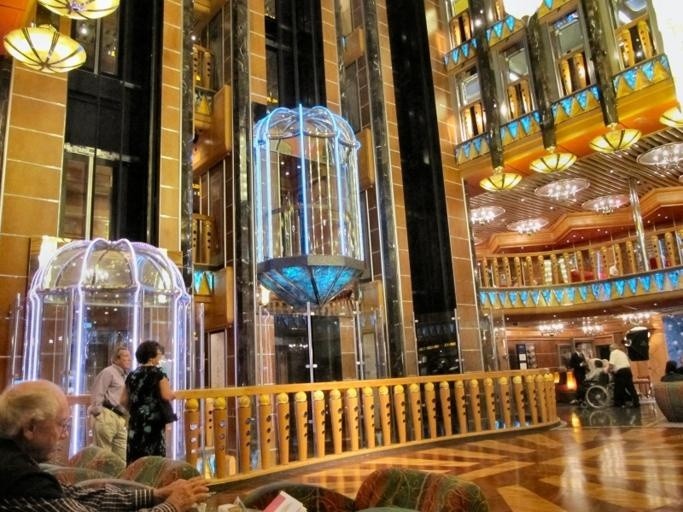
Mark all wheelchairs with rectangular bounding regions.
[579,371,614,411]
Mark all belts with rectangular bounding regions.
[101,401,125,417]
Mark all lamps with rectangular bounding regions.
[2,17,87,76]
[480,121,643,196]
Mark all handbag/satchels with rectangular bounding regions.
[153,370,178,424]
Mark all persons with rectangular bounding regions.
[84,343,131,466]
[569,341,589,405]
[583,353,607,374]
[0,380,211,512]
[608,340,639,410]
[119,341,179,471]
